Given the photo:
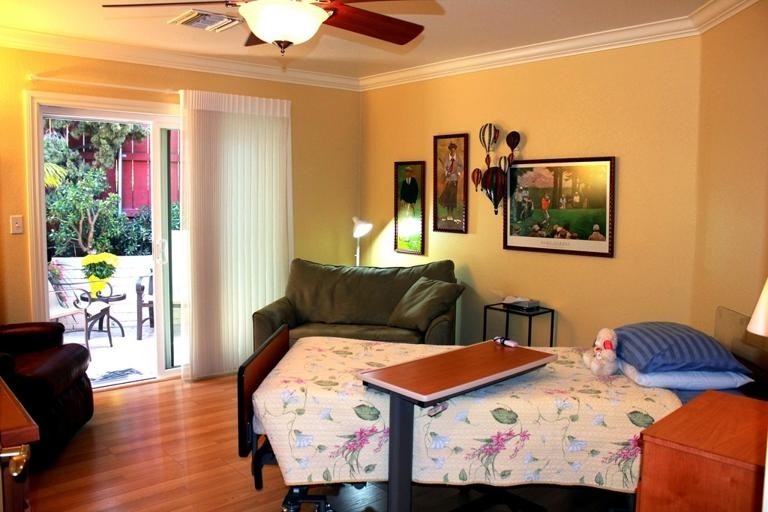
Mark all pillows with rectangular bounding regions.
[615,321,749,374]
[387,276,466,331]
[614,359,753,389]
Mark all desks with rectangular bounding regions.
[362,336,556,512]
[482,303,554,347]
[80,292,126,347]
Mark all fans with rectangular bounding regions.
[102,0,425,46]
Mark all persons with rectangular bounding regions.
[542,192,551,222]
[439,142,462,221]
[560,191,581,209]
[529,223,578,240]
[513,225,522,236]
[400,167,419,215]
[589,225,606,241]
[514,184,534,223]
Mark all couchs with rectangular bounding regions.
[252,258,457,352]
[0,322,93,469]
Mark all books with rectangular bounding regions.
[512,298,540,308]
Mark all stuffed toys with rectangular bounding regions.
[582,327,620,377]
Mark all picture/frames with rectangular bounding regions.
[503,156,615,259]
[433,133,469,234]
[394,161,425,255]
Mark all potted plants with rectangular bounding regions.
[81,252,118,294]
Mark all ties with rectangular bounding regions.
[448,159,455,172]
[407,178,410,185]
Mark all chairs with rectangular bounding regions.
[136,268,154,340]
[47,264,91,362]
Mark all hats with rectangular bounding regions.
[405,166,413,171]
[448,143,457,150]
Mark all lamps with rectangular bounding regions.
[239,1,334,55]
[747,278,768,339]
[352,215,373,266]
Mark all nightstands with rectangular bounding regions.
[636,389,765,511]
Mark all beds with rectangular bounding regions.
[235,322,765,509]
